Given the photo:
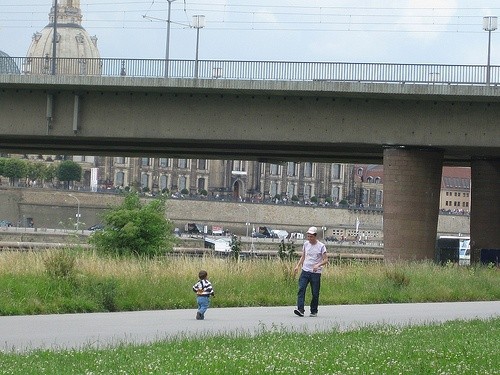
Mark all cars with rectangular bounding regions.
[88,223,105,231]
[0,219,13,228]
[250,231,267,238]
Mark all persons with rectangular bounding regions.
[192,270,216,320]
[294,226,329,316]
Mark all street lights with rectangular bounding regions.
[191,15,205,78]
[483,16,498,86]
[238,204,249,237]
[68,194,79,232]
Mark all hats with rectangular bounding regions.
[306,227,317,234]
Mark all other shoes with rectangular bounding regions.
[294,309,304,316]
[197,312,204,319]
[310,313,317,316]
